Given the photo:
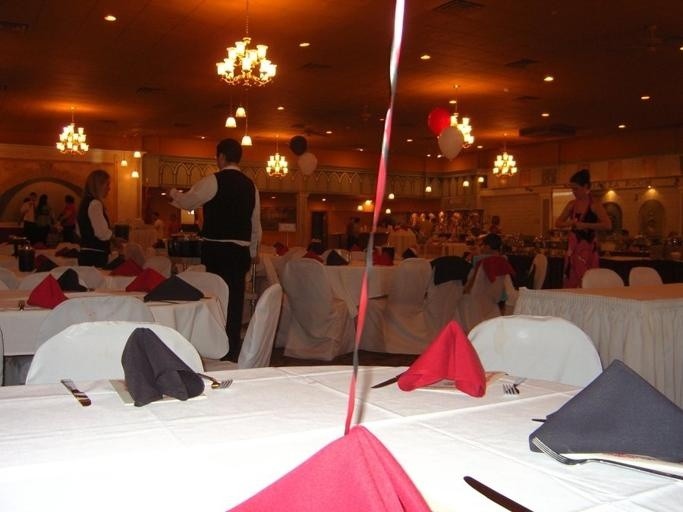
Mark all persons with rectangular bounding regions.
[74,169,127,269]
[459,213,515,317]
[345,215,355,250]
[149,211,165,247]
[271,240,289,257]
[553,167,612,289]
[430,218,439,237]
[300,240,324,265]
[417,216,432,244]
[163,138,261,365]
[54,194,78,229]
[16,192,39,241]
[162,212,177,240]
[33,193,54,244]
[350,217,363,251]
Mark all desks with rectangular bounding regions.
[1,367,682,512]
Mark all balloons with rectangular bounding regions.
[436,126,464,159]
[297,152,318,176]
[288,135,306,155]
[425,107,451,136]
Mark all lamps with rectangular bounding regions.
[216,0,278,88]
[492,136,518,177]
[437,85,475,149]
[120,151,141,178]
[56,107,90,157]
[266,136,289,178]
[225,90,252,146]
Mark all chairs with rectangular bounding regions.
[466,314,603,388]
[2,245,680,379]
[23,321,202,386]
